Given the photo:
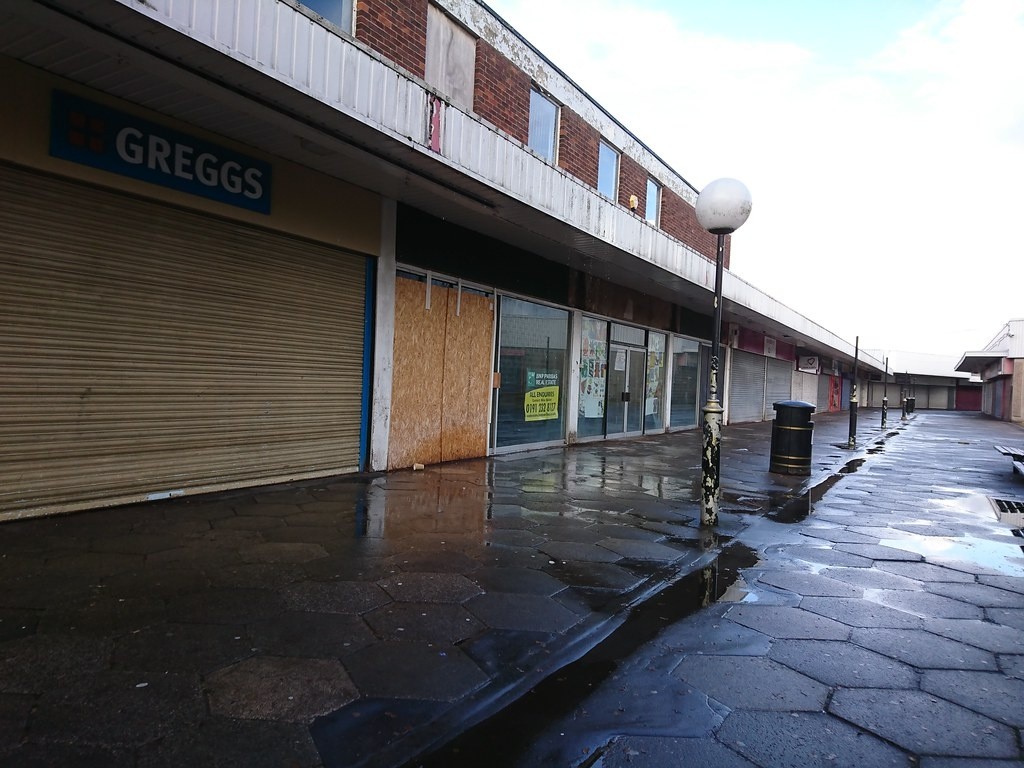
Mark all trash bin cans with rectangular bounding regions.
[767,398,819,476]
[906,397,916,415]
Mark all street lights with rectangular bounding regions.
[694,173,754,523]
